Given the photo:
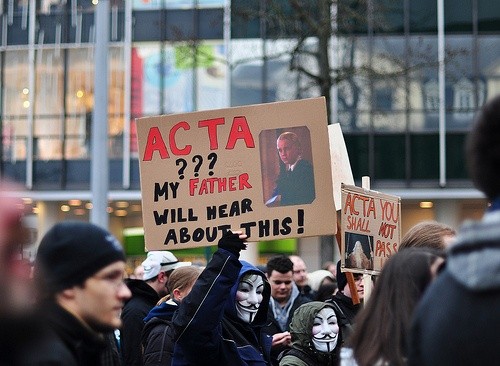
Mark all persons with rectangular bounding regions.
[262,255,313,366]
[0,221,133,366]
[171,230,275,366]
[279,301,343,366]
[349,241,370,270]
[288,255,311,303]
[340,248,445,366]
[143,265,204,366]
[398,221,459,258]
[266,132,315,207]
[326,260,364,347]
[406,92,500,366]
[306,261,340,300]
[119,251,179,366]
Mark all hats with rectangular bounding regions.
[147,250,192,273]
[33,223,126,297]
[336,258,375,291]
